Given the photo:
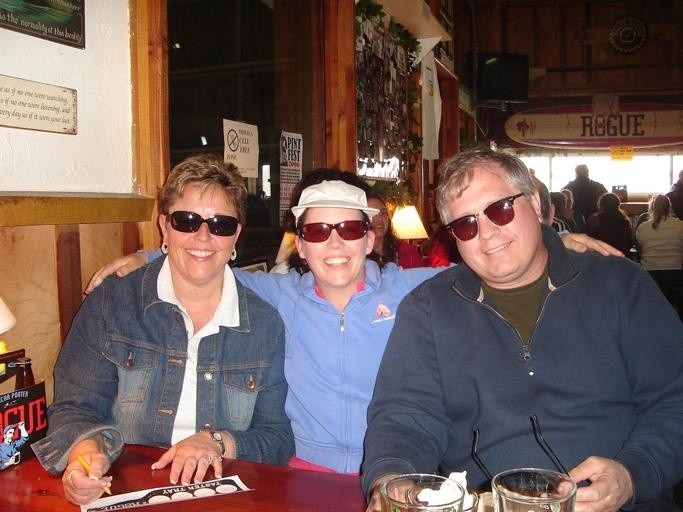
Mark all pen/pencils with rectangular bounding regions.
[77,455,112,496]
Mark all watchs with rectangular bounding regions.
[205,427,224,456]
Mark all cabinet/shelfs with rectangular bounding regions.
[400,50,462,259]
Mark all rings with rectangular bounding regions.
[65,470,76,490]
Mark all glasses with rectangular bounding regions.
[470,414,570,492]
[166,210,239,237]
[295,220,368,243]
[440,192,524,243]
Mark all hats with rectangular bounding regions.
[290,179,380,228]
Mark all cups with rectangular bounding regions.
[377,472,479,511]
[491,468,577,511]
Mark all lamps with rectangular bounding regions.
[390,201,430,253]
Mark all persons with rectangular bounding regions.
[359,145,683,511]
[585,191,635,258]
[530,175,555,227]
[423,217,462,267]
[529,169,535,175]
[559,165,608,220]
[665,171,682,220]
[366,193,399,272]
[46,152,296,506]
[269,208,311,275]
[634,194,682,319]
[549,189,585,234]
[83,164,627,477]
